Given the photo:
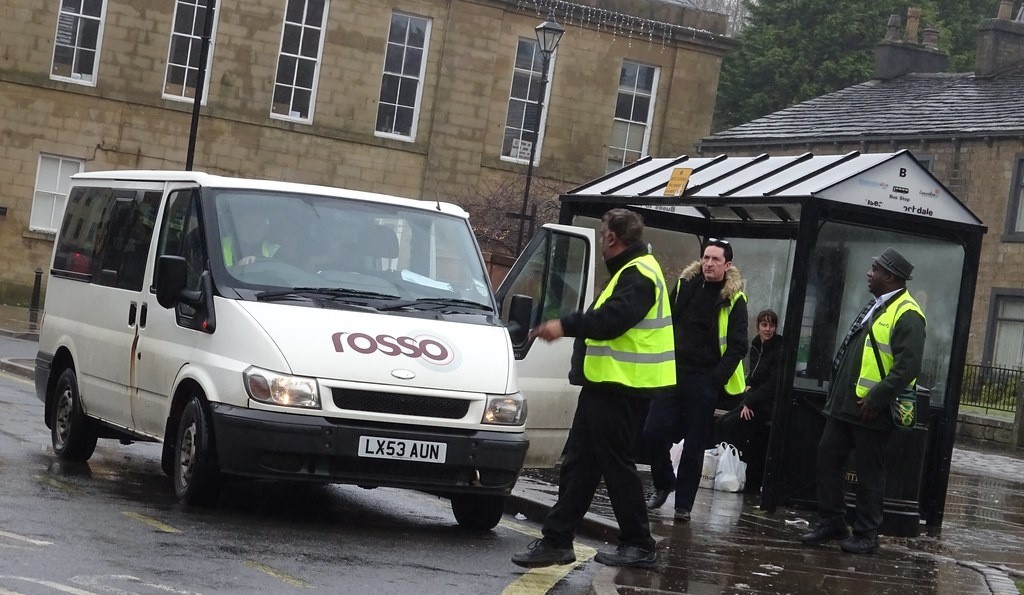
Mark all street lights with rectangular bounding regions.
[516,7,564,256]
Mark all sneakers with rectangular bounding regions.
[510,538,576,568]
[840,530,880,554]
[594,543,660,568]
[801,519,849,544]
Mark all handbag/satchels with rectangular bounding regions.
[699,441,747,492]
[887,387,918,431]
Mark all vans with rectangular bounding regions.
[34,170,597,530]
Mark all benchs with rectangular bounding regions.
[715,407,734,421]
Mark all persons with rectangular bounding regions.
[646,239,749,521]
[799,247,925,553]
[511,207,676,572]
[224,199,294,268]
[711,310,781,483]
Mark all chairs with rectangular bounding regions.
[357,225,399,276]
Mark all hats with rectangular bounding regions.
[872,246,915,280]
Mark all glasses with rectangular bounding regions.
[709,237,729,244]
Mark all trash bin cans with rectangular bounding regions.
[878,383,932,537]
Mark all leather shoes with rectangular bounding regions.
[647,487,671,508]
[675,507,691,521]
